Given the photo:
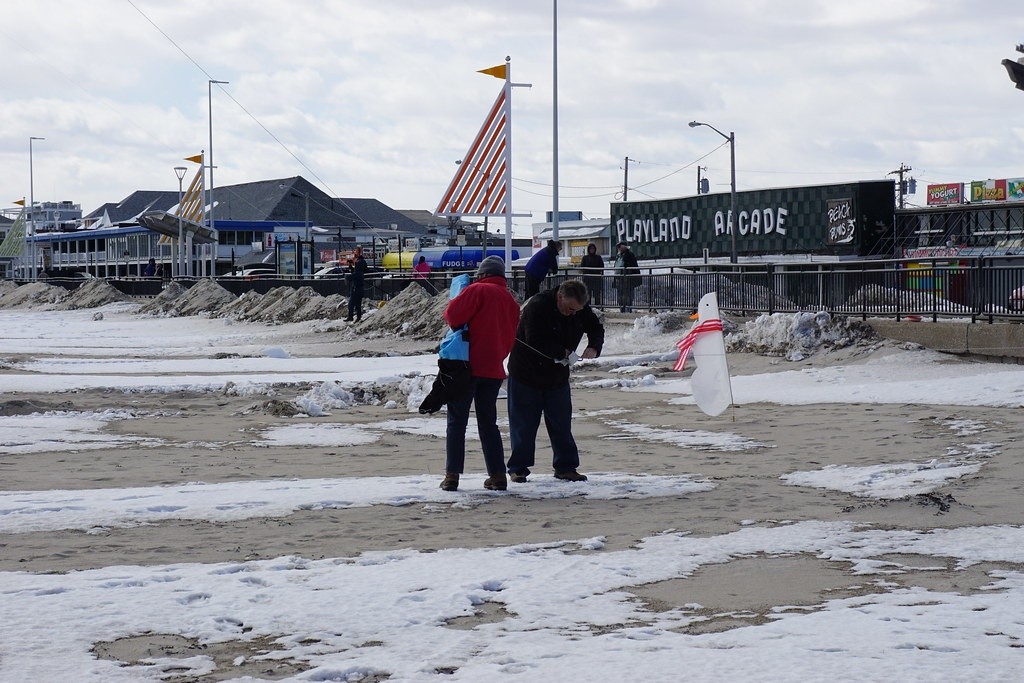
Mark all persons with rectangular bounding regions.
[524,239,563,300]
[507,280,605,479]
[581,244,604,312]
[343,263,365,324]
[413,256,432,287]
[144,258,163,280]
[440,256,521,491]
[613,241,643,313]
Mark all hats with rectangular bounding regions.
[477,255,505,277]
[616,240,627,246]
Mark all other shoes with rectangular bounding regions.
[344,318,353,322]
[353,317,362,324]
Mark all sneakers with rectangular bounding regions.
[554,467,587,482]
[440,471,459,490]
[484,471,507,490]
[511,471,527,483]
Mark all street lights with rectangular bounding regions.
[454,160,490,263]
[31,136,45,283]
[208,81,230,285]
[687,120,739,276]
[174,166,188,277]
[279,183,309,240]
